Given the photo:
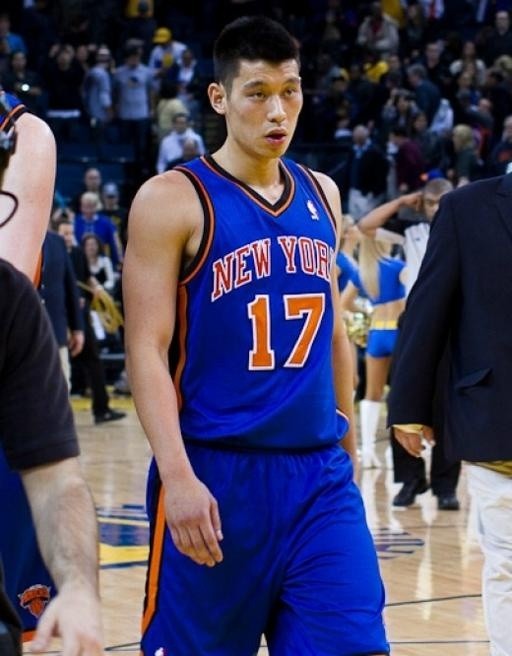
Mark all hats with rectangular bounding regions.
[153,27,171,42]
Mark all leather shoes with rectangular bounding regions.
[438,493,459,510]
[394,479,428,506]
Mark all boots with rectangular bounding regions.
[359,399,382,468]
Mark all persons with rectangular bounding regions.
[355,174,467,509]
[1,91,84,645]
[112,13,399,656]
[335,222,430,476]
[2,28,207,427]
[1,258,116,656]
[291,1,511,402]
[380,153,509,655]
[359,468,488,656]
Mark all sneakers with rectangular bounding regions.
[95,409,125,423]
